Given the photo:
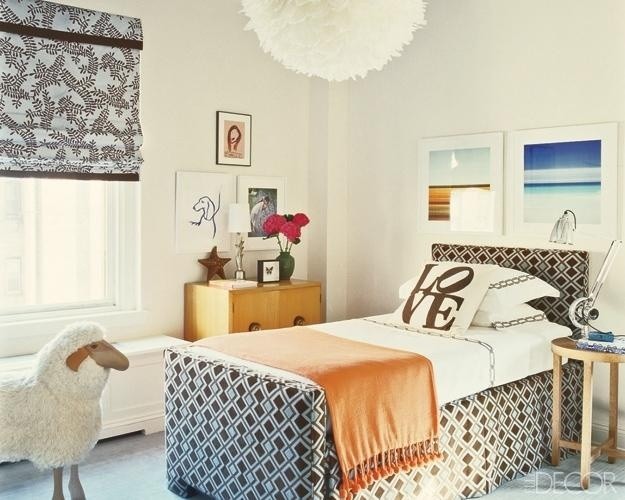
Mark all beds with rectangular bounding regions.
[163,244,589,500]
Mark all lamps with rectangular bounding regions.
[228,203,252,280]
[548,210,623,341]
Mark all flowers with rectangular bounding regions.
[262,213,310,252]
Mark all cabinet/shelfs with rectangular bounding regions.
[0,333,194,442]
[184,277,323,343]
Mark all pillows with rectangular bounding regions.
[388,261,560,340]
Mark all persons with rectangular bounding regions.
[249,194,272,234]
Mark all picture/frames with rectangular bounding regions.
[216,111,252,166]
[419,132,504,237]
[511,123,618,238]
[237,176,286,251]
[175,172,234,253]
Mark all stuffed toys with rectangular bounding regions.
[0,320,130,499]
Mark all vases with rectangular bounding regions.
[275,252,295,280]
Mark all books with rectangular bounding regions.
[208,277,259,290]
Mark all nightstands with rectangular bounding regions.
[551,335,625,490]
